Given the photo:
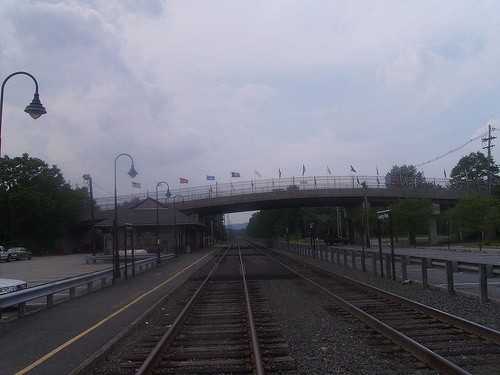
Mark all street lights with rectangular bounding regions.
[82,174,96,263]
[114,154,137,277]
[0,71,47,160]
[174,194,184,257]
[361,181,371,248]
[156,181,172,264]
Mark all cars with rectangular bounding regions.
[7,247,32,261]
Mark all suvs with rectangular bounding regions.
[324,235,348,245]
[0,246,12,262]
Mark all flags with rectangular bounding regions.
[132,182,141,188]
[180,178,188,184]
[231,172,241,177]
[207,175,215,180]
